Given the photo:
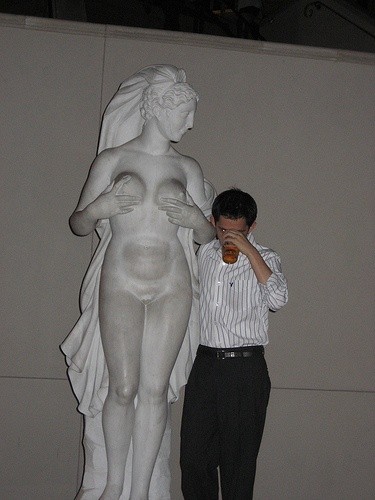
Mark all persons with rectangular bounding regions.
[56,65,216,500]
[176,186,291,500]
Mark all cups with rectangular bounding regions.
[222,236,240,264]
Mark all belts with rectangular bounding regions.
[196,346,265,358]
